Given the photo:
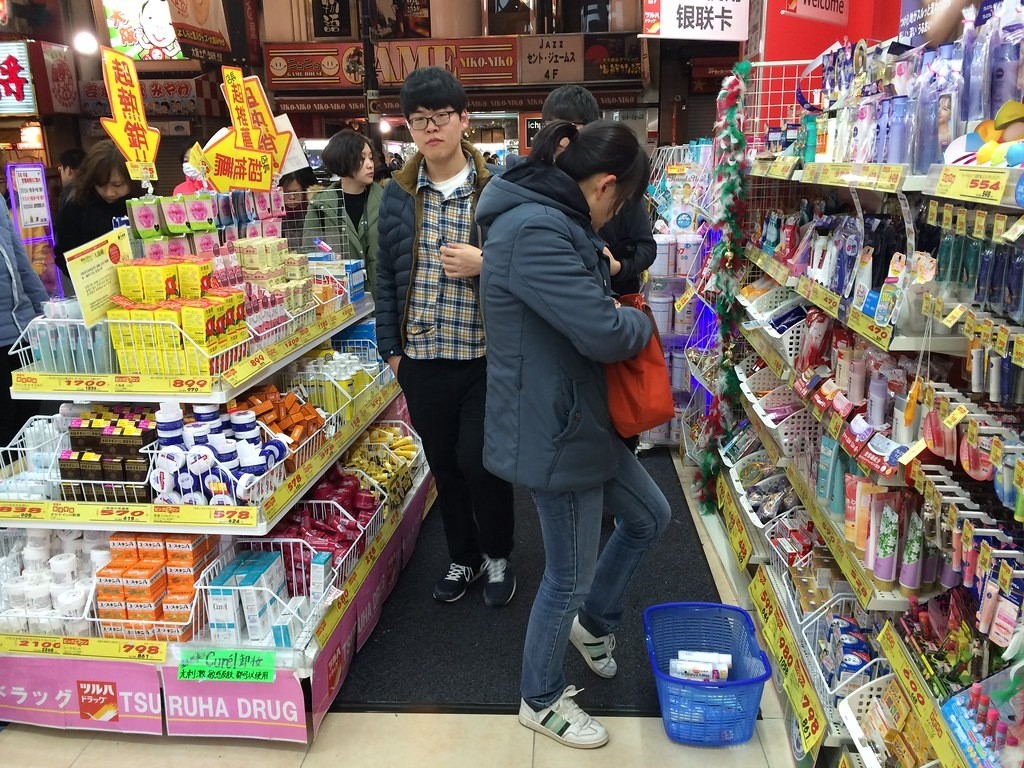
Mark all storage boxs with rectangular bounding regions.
[95,532,332,650]
[58,403,161,502]
[106,236,365,375]
[219,384,326,477]
[769,511,938,768]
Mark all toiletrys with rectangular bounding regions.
[668,649,733,679]
[717,198,963,710]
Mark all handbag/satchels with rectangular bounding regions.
[599,291,676,440]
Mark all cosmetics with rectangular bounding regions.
[0,236,419,647]
[667,650,742,722]
[787,0,1024,768]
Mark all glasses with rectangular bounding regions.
[57,166,67,173]
[408,109,461,131]
[543,119,584,131]
[283,190,304,198]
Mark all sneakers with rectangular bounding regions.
[479,554,517,610]
[432,562,486,603]
[568,613,618,679]
[518,684,610,749]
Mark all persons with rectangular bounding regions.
[376,68,516,605]
[58,149,86,186]
[278,173,308,239]
[483,152,501,165]
[474,120,671,749]
[386,152,406,171]
[299,131,384,301]
[0,195,63,467]
[54,138,159,279]
[541,85,657,527]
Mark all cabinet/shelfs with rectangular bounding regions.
[717,151,967,567]
[0,292,432,688]
[750,160,1024,746]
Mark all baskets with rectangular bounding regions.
[641,602,773,748]
